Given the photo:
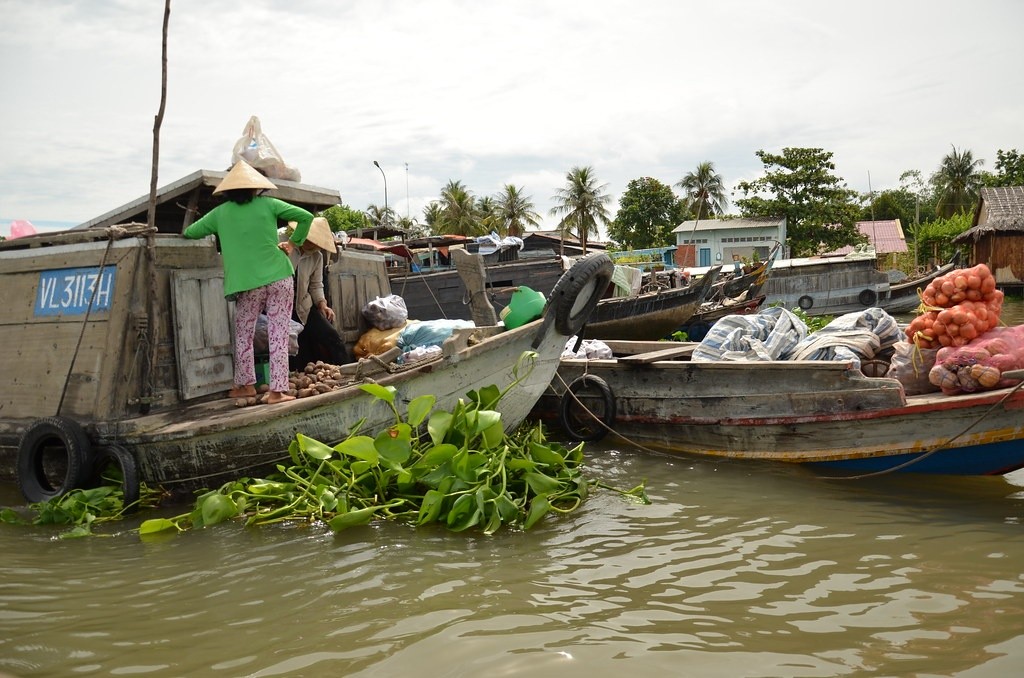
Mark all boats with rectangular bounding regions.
[1,221,613,518]
[340,223,1024,476]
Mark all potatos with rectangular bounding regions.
[235,361,344,407]
[904,263,1005,345]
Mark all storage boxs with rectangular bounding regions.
[253,363,269,387]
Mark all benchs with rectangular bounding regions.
[617,345,698,364]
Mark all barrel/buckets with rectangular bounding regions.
[492,285,547,330]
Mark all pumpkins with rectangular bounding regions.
[928,324,1024,395]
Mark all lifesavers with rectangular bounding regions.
[540,248,616,338]
[859,288,877,306]
[16,412,96,501]
[558,372,618,442]
[797,294,813,311]
[90,441,141,520]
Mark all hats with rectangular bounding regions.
[212,160,278,195]
[287,217,337,254]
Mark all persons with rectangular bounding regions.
[182,159,314,403]
[289,216,350,373]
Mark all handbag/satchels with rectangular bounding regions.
[362,292,407,331]
